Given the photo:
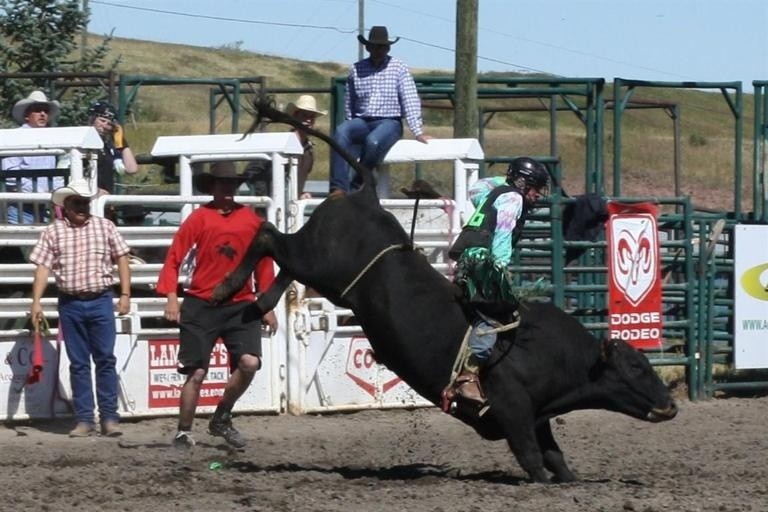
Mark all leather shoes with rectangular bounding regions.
[69,417,123,440]
[453,365,487,405]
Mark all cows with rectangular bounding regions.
[206,77,679,484]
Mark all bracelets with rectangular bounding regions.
[120,293,131,297]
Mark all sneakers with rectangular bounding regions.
[207,412,248,450]
[329,188,346,201]
[172,427,194,452]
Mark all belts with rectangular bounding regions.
[64,292,108,300]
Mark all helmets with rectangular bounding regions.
[87,102,117,136]
[507,157,550,203]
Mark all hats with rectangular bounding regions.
[400,178,442,201]
[51,176,98,207]
[286,94,327,121]
[357,25,399,47]
[193,158,252,195]
[12,90,59,124]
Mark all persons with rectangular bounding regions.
[32,179,131,435]
[83,101,138,225]
[448,157,548,405]
[329,26,434,198]
[244,94,329,218]
[157,162,278,448]
[1,90,68,263]
